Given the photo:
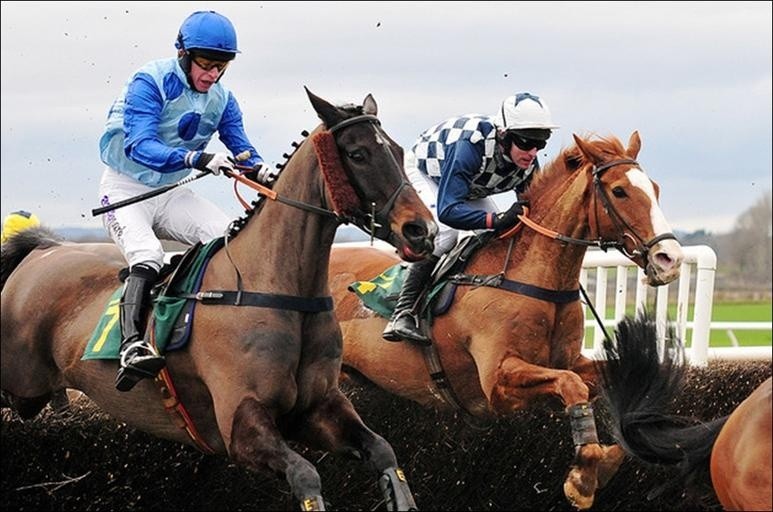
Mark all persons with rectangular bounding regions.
[383,92,560,347]
[98,10,272,392]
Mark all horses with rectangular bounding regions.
[326,131,684,510]
[593,304,773,512]
[0,85,439,512]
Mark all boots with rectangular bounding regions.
[114,277,166,392]
[382,254,441,347]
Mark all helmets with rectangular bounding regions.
[174,11,241,72]
[493,93,560,148]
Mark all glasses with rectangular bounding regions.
[192,56,228,72]
[512,135,547,151]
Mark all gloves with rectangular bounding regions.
[244,162,275,184]
[185,151,236,178]
[492,200,530,229]
